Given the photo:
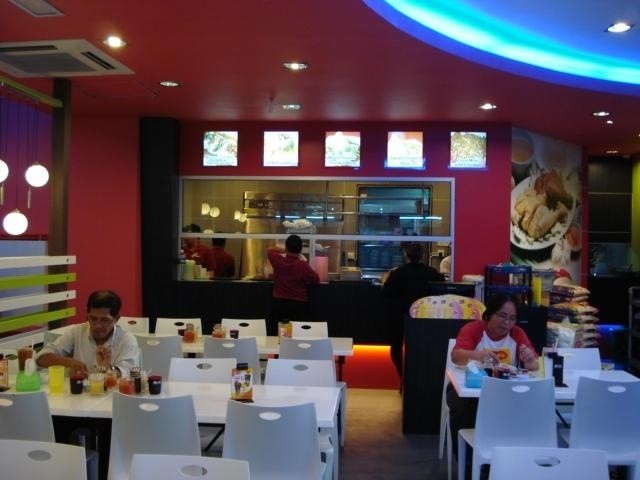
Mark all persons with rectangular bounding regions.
[207,231,235,277]
[34,290,140,379]
[182,224,210,271]
[439,241,452,281]
[446,292,540,480]
[267,234,320,336]
[381,243,445,377]
[590,247,601,267]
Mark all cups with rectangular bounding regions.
[184,260,207,281]
[543,356,553,380]
[212,324,227,339]
[493,363,511,378]
[70,365,134,396]
[16,346,34,372]
[552,355,564,388]
[148,375,162,395]
[511,129,538,176]
[48,365,66,398]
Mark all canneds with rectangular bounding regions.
[95,367,107,391]
[118,377,136,396]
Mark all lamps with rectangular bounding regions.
[3,97,29,237]
[24,100,49,188]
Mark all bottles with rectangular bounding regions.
[277,318,292,344]
[182,323,195,342]
[129,366,147,394]
[483,357,493,376]
[0,354,10,388]
[229,322,240,340]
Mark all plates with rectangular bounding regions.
[328,266,361,281]
[359,249,403,269]
[510,168,577,251]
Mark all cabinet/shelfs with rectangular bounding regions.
[628,286,639,375]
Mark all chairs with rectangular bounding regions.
[0,316,640,479]
